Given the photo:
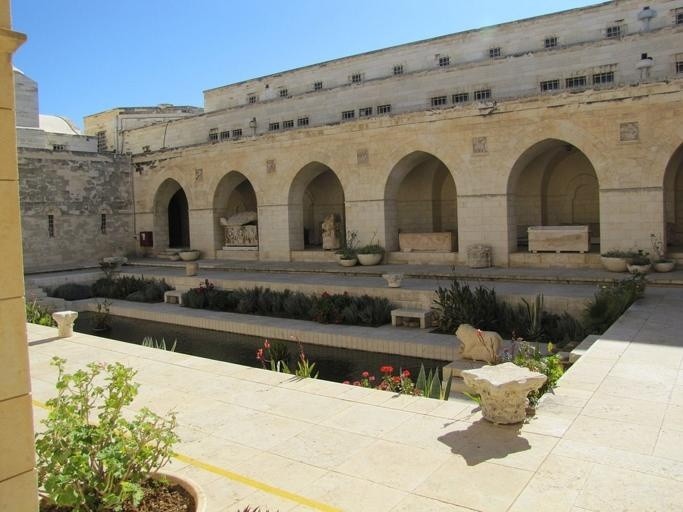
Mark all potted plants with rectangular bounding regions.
[170,250,201,261]
[31,356,206,512]
[601,248,677,276]
[334,231,385,267]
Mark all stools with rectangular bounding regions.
[389,306,434,329]
[163,289,188,306]
